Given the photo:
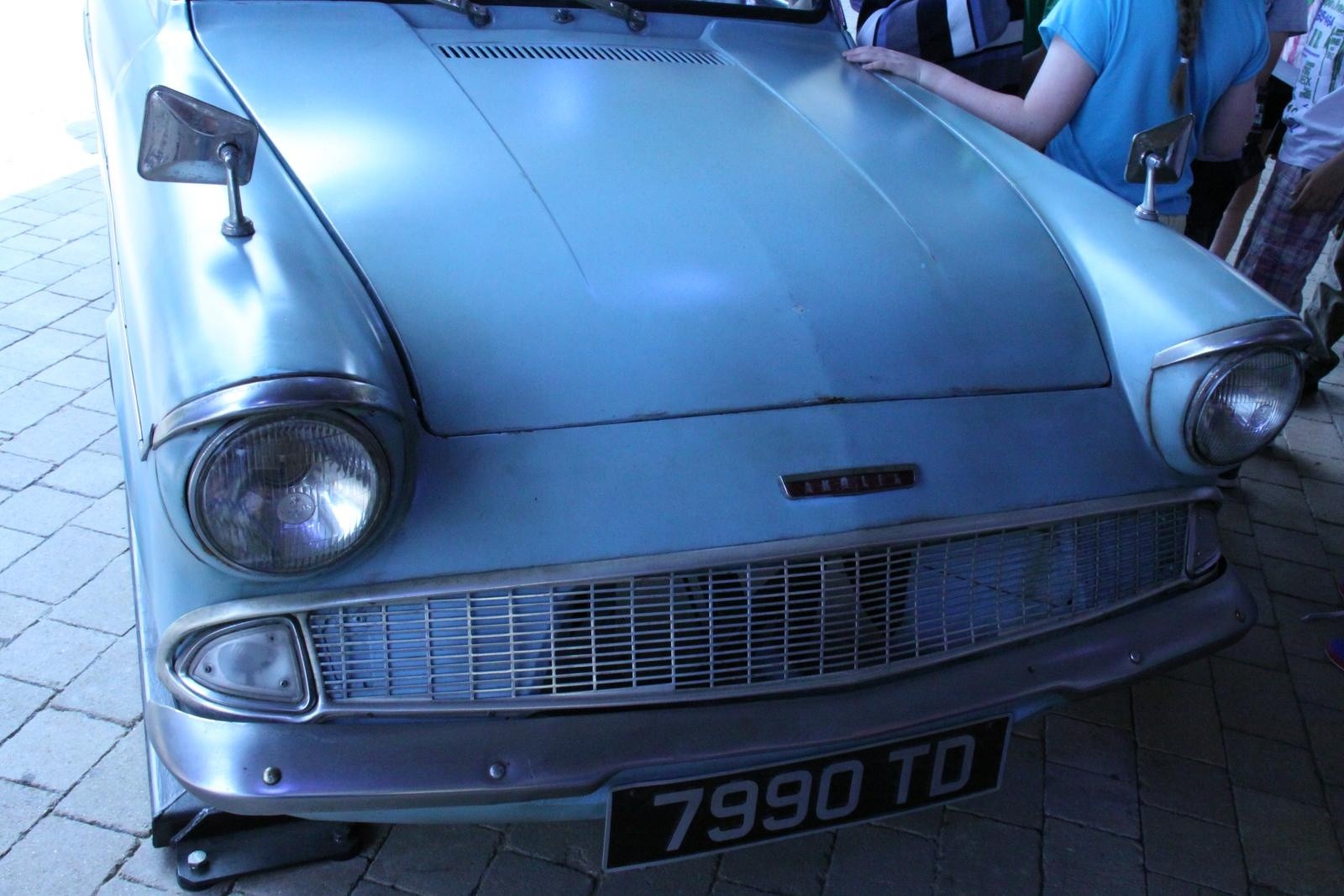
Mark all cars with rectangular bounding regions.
[78,1,1342,889]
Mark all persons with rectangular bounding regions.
[842,0,1344,399]
